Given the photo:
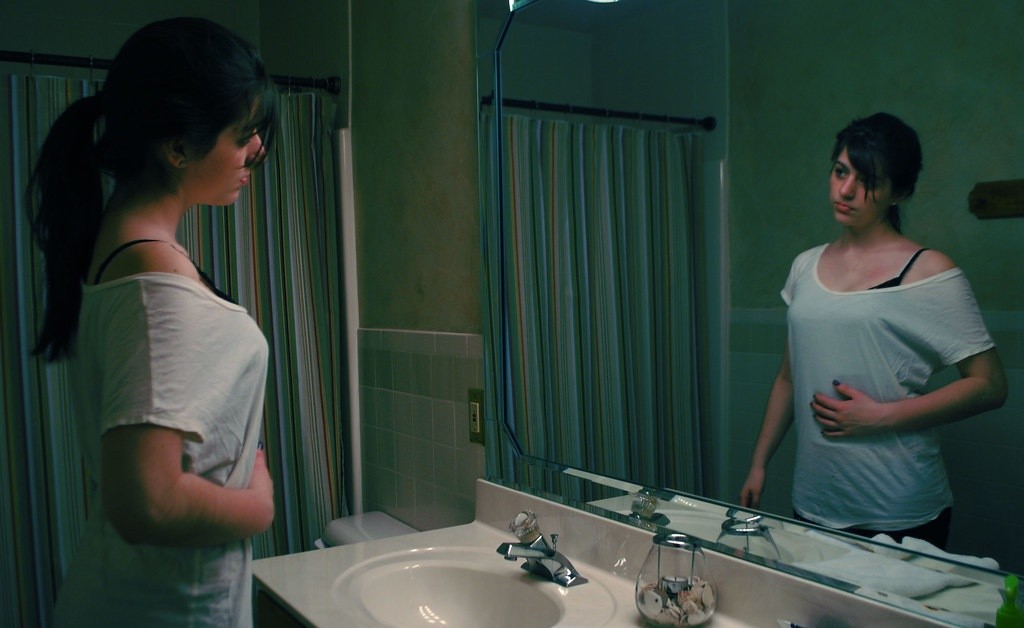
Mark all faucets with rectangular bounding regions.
[627,493,672,527]
[496,508,588,587]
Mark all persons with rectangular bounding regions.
[22,17,281,627]
[739,113,1009,551]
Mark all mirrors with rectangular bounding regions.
[474,0,1024,628]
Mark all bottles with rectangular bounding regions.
[635,533,717,628]
[716,508,781,561]
[996,574,1024,628]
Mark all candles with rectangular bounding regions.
[662,577,687,593]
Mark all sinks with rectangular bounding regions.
[618,507,794,565]
[359,563,564,628]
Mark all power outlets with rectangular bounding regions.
[469,402,480,433]
